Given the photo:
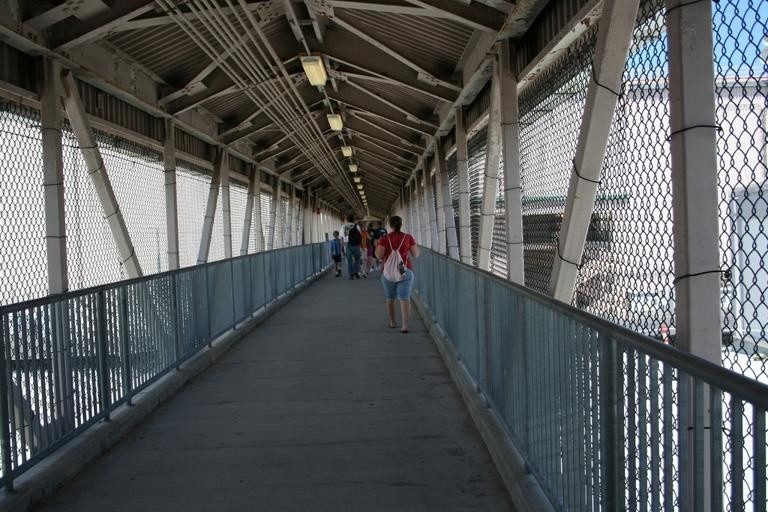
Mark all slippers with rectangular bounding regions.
[388,321,409,333]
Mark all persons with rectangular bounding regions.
[374,216,421,334]
[329,214,385,281]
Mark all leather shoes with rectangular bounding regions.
[348,273,360,281]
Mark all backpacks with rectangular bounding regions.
[383,232,408,283]
[347,223,362,247]
